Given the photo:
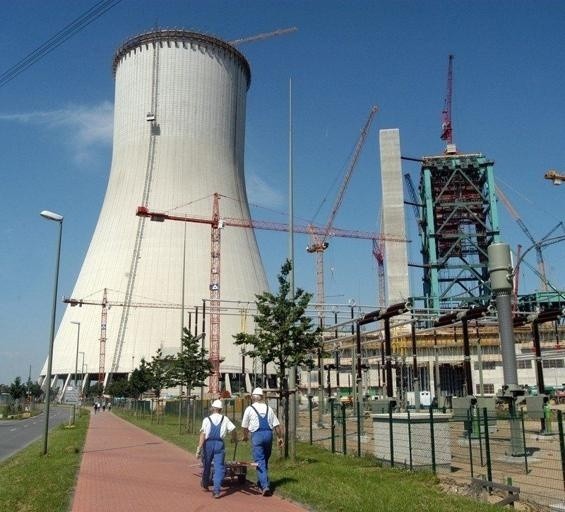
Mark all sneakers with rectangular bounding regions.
[201,482,272,498]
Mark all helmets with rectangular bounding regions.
[251,386,264,396]
[212,400,223,409]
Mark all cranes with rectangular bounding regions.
[543,167,565,185]
[404,173,422,234]
[440,53,459,155]
[60,287,228,400]
[305,102,382,331]
[494,176,565,294]
[372,236,385,312]
[133,192,413,400]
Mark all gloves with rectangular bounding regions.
[195,446,201,460]
[276,438,285,448]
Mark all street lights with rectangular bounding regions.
[39,210,64,457]
[69,320,82,429]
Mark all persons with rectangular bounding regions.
[240,387,285,496]
[92,400,111,414]
[195,400,237,498]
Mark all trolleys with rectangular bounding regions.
[191,438,248,489]
